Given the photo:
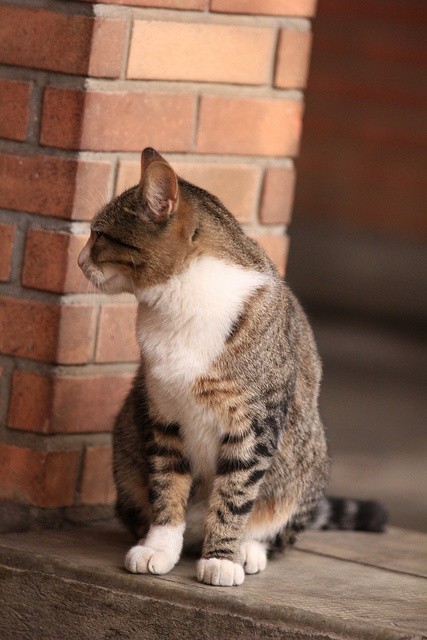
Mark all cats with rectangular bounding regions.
[75,146,387,586]
